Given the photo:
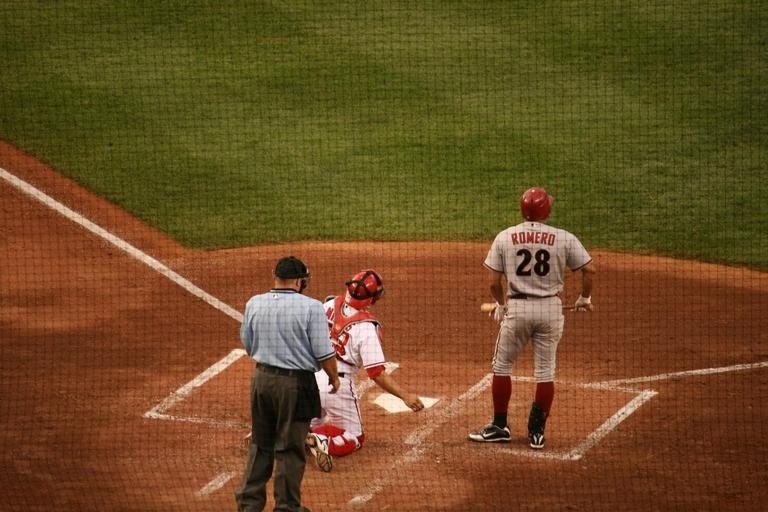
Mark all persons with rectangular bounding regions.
[466,186,596,450]
[233,254,341,512]
[242,267,425,472]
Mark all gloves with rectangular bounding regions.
[489,302,508,322]
[575,294,592,312]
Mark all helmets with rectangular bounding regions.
[344,267,385,308]
[519,187,554,221]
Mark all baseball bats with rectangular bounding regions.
[480,303,591,311]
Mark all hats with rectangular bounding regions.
[274,255,309,280]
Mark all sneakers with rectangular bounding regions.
[527,428,545,450]
[306,433,333,473]
[467,424,511,443]
[242,432,253,449]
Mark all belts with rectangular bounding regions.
[338,373,344,377]
[255,362,304,376]
[507,291,559,299]
